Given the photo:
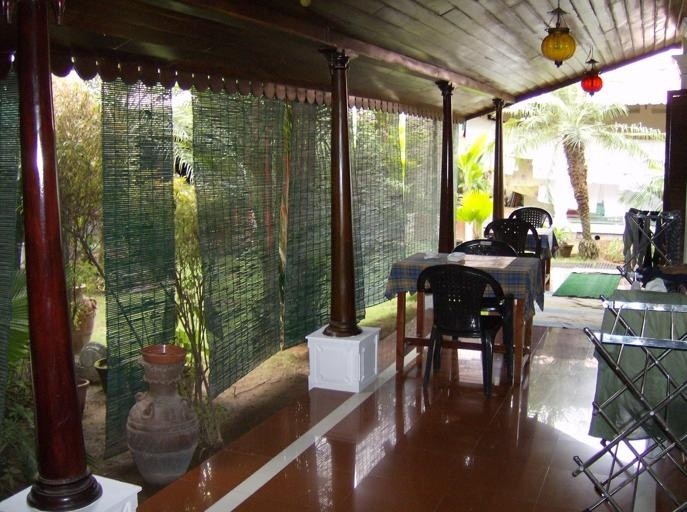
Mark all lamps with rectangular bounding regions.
[581,46,603,95]
[541,0,576,67]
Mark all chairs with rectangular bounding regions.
[508,207,552,228]
[451,239,519,362]
[484,219,541,257]
[416,264,513,398]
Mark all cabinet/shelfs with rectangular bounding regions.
[617,208,678,292]
[572,288,687,512]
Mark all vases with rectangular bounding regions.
[125,345,201,485]
[93,357,108,393]
[75,376,90,416]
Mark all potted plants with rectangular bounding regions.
[18,84,101,356]
[554,229,574,257]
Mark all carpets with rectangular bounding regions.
[553,272,622,299]
[533,290,603,331]
